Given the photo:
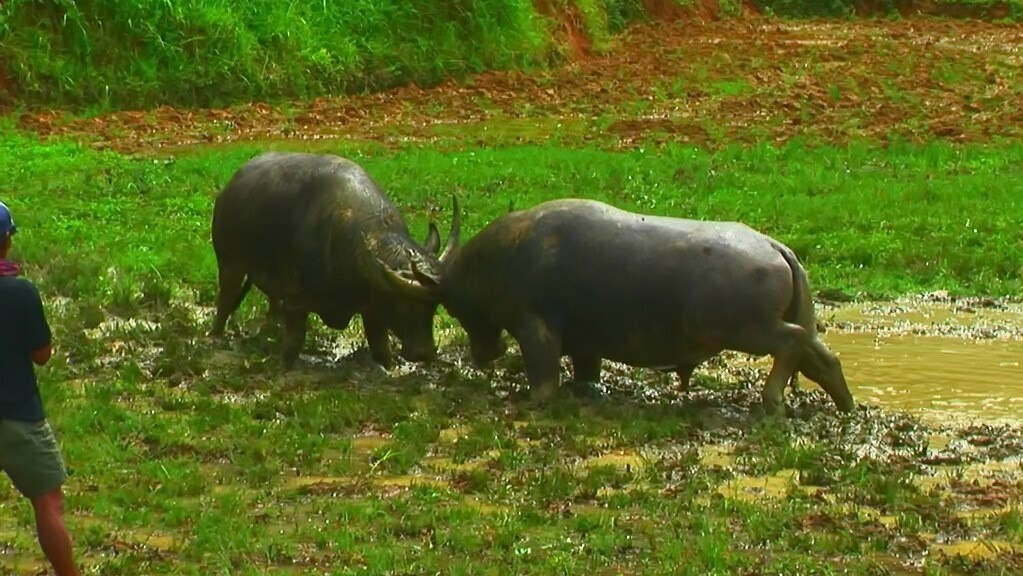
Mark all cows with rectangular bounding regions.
[405,198,861,438]
[206,149,463,381]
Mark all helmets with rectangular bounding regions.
[0,202,17,235]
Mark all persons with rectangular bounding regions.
[0,201,81,576]
